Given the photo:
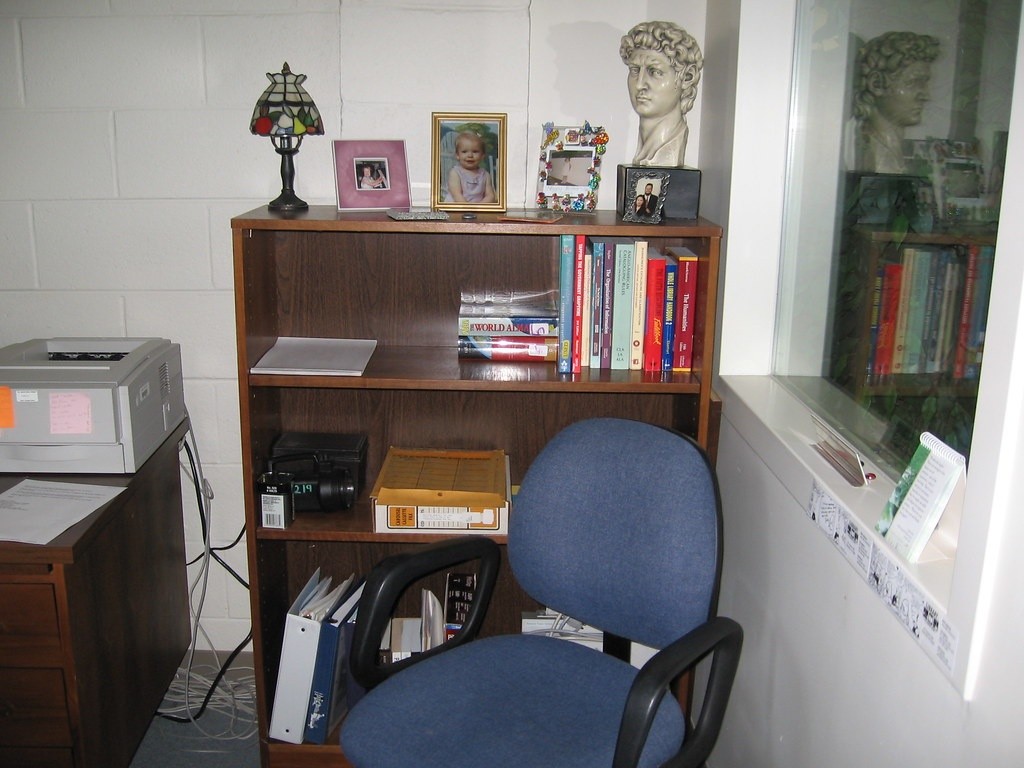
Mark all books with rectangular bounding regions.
[864,244,994,377]
[456,292,559,362]
[377,589,446,663]
[559,233,698,374]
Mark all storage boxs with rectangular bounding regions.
[368,445,514,537]
[274,429,372,494]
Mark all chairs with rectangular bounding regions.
[340,413,745,768]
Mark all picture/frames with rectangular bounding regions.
[532,121,609,216]
[430,112,508,213]
[913,131,1009,224]
[622,169,672,223]
[331,139,412,212]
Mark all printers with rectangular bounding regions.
[1,336,187,475]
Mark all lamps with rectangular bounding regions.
[249,61,326,212]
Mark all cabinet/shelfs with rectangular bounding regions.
[232,200,724,768]
[842,221,995,456]
[0,418,192,768]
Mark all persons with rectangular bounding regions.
[852,32,939,175]
[560,157,571,184]
[643,183,658,213]
[443,133,495,203]
[618,20,703,166]
[635,195,651,216]
[361,166,383,189]
